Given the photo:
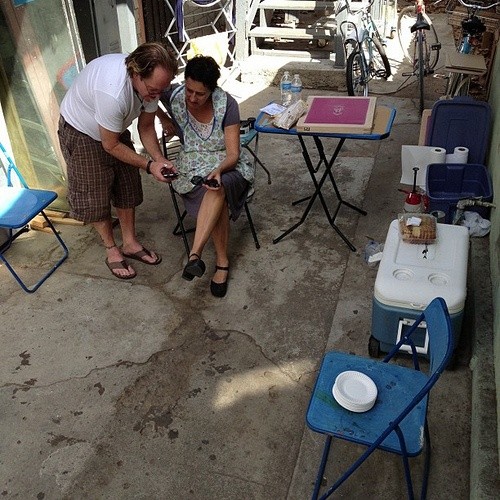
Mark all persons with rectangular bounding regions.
[58,42,178,279]
[155,55,256,297]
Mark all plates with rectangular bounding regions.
[332,371,378,412]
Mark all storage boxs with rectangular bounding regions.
[368,219,469,371]
[425,163,492,225]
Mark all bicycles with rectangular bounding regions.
[396,0,441,115]
[336,0,392,98]
[433,0,500,98]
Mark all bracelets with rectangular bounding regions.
[147,160,153,174]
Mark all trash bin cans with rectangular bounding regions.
[424,163,492,222]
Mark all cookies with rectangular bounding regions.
[402,215,436,243]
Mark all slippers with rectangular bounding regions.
[105,256,136,279]
[123,246,162,265]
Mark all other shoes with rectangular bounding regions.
[211,265,229,298]
[182,254,205,281]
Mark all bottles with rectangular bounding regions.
[291,74,302,105]
[280,71,291,106]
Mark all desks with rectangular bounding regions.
[253,100,395,253]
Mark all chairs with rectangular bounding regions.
[0,143,69,293]
[305,296,454,500]
[162,83,272,260]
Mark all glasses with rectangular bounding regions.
[190,176,220,188]
[142,76,172,97]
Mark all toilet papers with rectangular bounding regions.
[399,144,447,187]
[446,146,469,164]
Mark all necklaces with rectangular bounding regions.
[135,90,146,113]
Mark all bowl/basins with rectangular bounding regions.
[399,213,439,244]
